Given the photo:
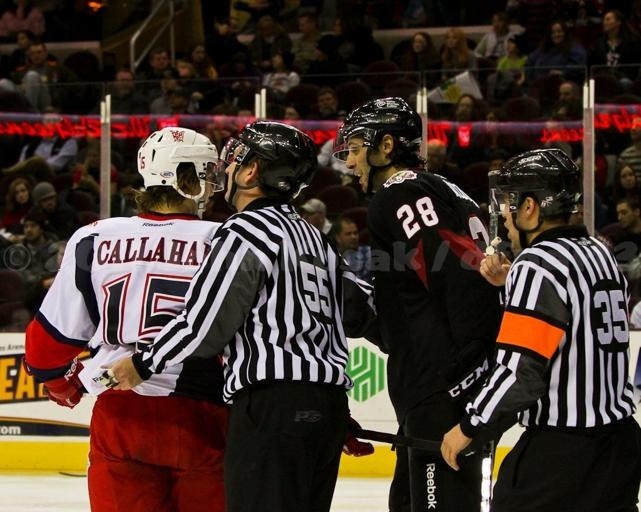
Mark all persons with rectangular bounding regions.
[3,3,640,511]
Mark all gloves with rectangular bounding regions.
[341,415,375,458]
[40,355,87,410]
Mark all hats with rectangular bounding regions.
[24,211,48,230]
[32,181,57,206]
[298,198,327,215]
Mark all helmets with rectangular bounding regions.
[136,126,219,205]
[487,146,584,218]
[219,119,321,206]
[331,96,426,168]
